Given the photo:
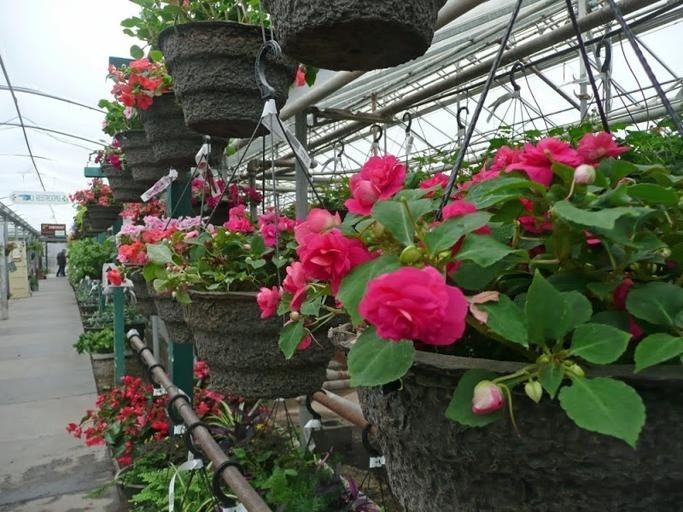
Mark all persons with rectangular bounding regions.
[55,249,68,277]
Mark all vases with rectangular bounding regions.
[101,163,154,203]
[314,418,355,452]
[180,287,347,398]
[139,91,229,169]
[263,0,447,72]
[74,206,106,233]
[157,22,299,136]
[85,203,124,229]
[80,304,100,315]
[115,129,170,180]
[152,290,197,346]
[338,339,683,512]
[203,199,232,226]
[129,272,158,316]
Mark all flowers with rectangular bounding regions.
[93,99,142,134]
[105,57,173,108]
[86,138,131,173]
[257,131,682,451]
[106,215,215,286]
[120,1,318,87]
[66,362,223,479]
[67,178,116,207]
[191,178,262,208]
[144,205,302,305]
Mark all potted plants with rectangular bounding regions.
[73,329,128,354]
[83,303,148,341]
[82,452,167,509]
